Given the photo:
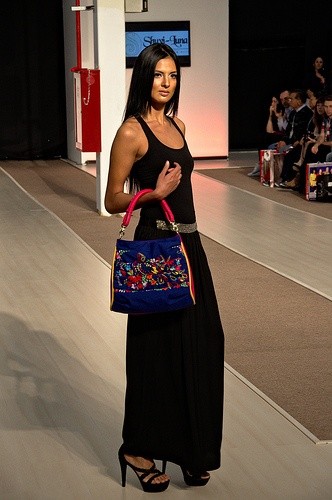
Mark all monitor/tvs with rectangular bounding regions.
[126,21,191,69]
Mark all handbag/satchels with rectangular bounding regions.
[305,161,332,201]
[110,189,196,315]
[317,172,332,201]
[270,146,294,187]
[260,150,278,183]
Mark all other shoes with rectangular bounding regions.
[286,178,300,187]
[293,162,302,171]
[248,166,260,176]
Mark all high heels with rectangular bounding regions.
[161,459,210,486]
[118,443,170,492]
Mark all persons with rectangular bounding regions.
[248,85,332,188]
[310,167,332,201]
[310,57,328,95]
[105,43,225,492]
